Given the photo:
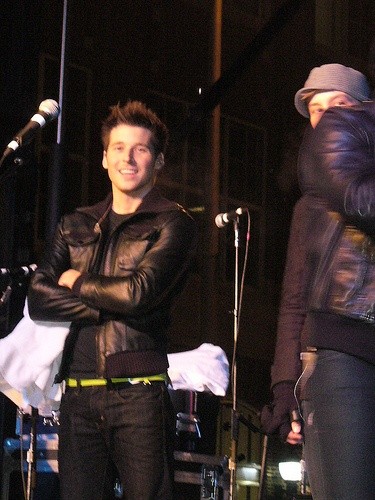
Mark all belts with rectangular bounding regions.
[66,373,166,387]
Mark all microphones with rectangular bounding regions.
[1,264,38,277]
[215,207,247,228]
[4,99,60,156]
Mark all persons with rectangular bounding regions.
[260,64,375,500]
[28,100,200,500]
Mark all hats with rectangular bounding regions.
[294,64,370,119]
